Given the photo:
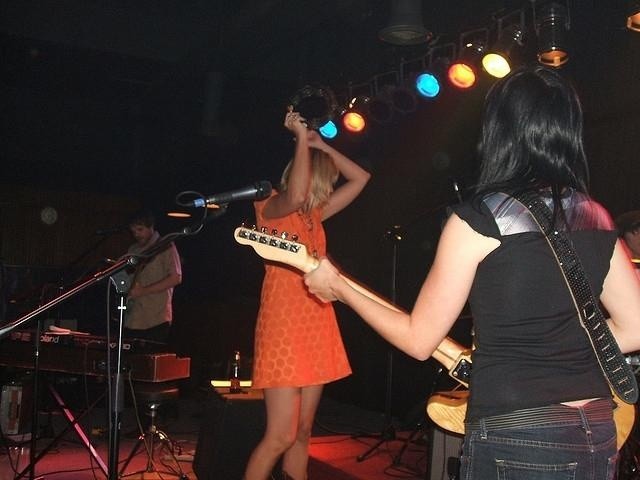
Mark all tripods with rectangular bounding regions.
[393,365,437,467]
[354,242,425,462]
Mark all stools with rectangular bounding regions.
[117,382,190,480]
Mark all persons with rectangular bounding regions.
[614,209,640,273]
[122,216,182,439]
[244,111,372,480]
[304,65,640,480]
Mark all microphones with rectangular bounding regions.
[194,181,272,207]
[95,225,129,235]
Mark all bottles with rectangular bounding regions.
[228,351,243,393]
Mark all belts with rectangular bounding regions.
[466,399,614,433]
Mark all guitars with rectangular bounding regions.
[234,222,637,456]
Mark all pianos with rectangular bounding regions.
[1,326,191,384]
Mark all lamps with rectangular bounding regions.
[314,1,574,140]
